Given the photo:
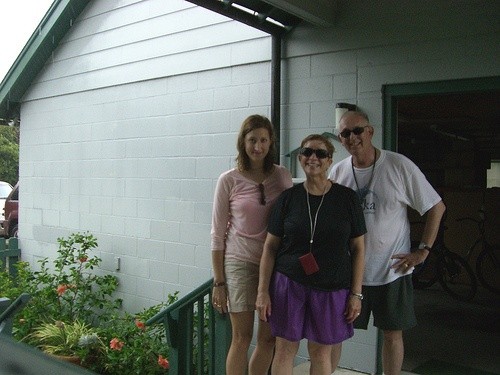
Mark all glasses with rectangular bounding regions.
[339,126,369,138]
[259,183,267,206]
[299,147,332,158]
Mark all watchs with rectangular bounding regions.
[418,242,432,252]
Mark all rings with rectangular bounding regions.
[214,302,221,308]
[357,312,361,316]
[404,263,409,268]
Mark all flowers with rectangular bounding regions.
[19,318,96,355]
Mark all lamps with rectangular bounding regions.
[7,116,21,127]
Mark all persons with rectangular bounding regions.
[210,114,293,375]
[255,134,368,375]
[326,110,446,375]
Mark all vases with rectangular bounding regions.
[57,354,80,363]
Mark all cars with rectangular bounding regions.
[0,180,14,220]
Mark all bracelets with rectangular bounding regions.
[212,281,226,287]
[352,289,364,300]
